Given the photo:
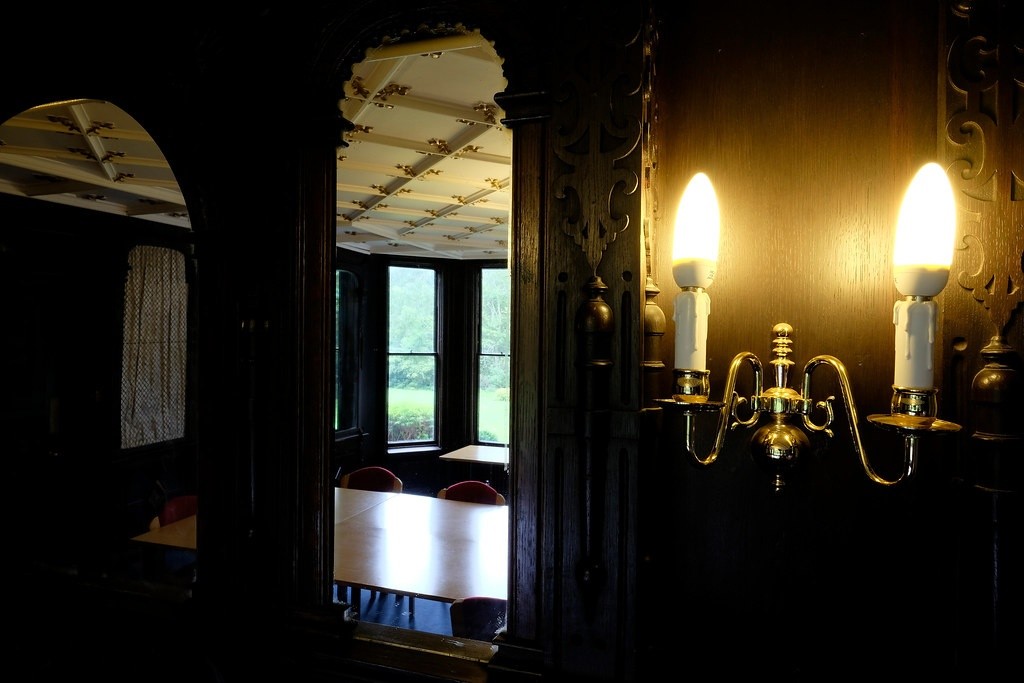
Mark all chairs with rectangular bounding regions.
[450,594,508,641]
[436,480,505,508]
[342,466,403,600]
[157,493,195,527]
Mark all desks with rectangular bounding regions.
[439,445,511,469]
[133,486,511,636]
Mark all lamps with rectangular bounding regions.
[659,161,965,493]
